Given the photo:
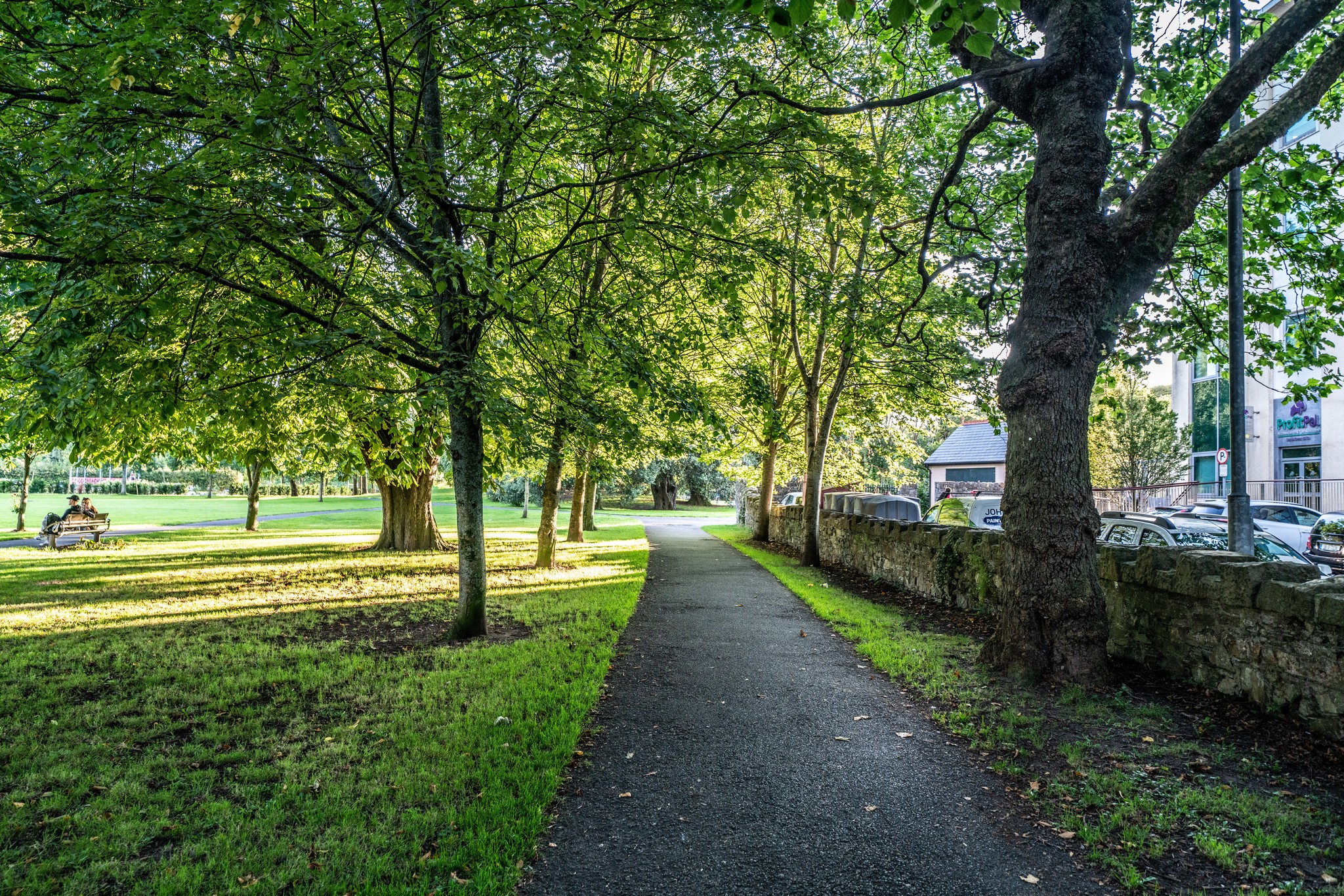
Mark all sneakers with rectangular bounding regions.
[40,539,49,546]
[34,535,45,540]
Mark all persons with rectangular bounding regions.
[943,487,953,499]
[77,497,100,531]
[34,495,95,546]
[970,489,979,496]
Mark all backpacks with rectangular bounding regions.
[47,512,64,534]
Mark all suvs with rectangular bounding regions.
[921,490,1005,530]
[1096,506,1316,566]
[1139,503,1196,516]
[1190,499,1324,556]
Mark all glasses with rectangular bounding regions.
[83,497,89,500]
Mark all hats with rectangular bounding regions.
[970,490,978,493]
[66,495,79,502]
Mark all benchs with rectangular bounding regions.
[47,513,111,549]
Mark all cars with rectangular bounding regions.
[1301,509,1343,575]
[777,492,803,505]
